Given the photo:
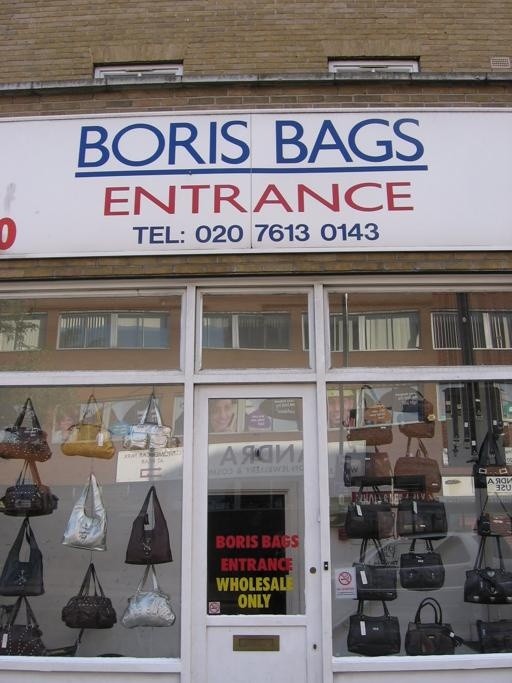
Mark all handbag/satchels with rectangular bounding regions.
[61,562,118,630]
[464,534,512,605]
[62,472,108,553]
[464,618,512,654]
[0,516,45,597]
[0,455,58,519]
[121,393,173,453]
[476,491,512,538]
[121,564,176,629]
[60,394,116,460]
[0,595,45,657]
[125,485,174,566]
[473,430,512,491]
[0,397,53,462]
[342,384,464,656]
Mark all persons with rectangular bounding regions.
[53,404,80,444]
[209,397,239,435]
[327,388,355,429]
